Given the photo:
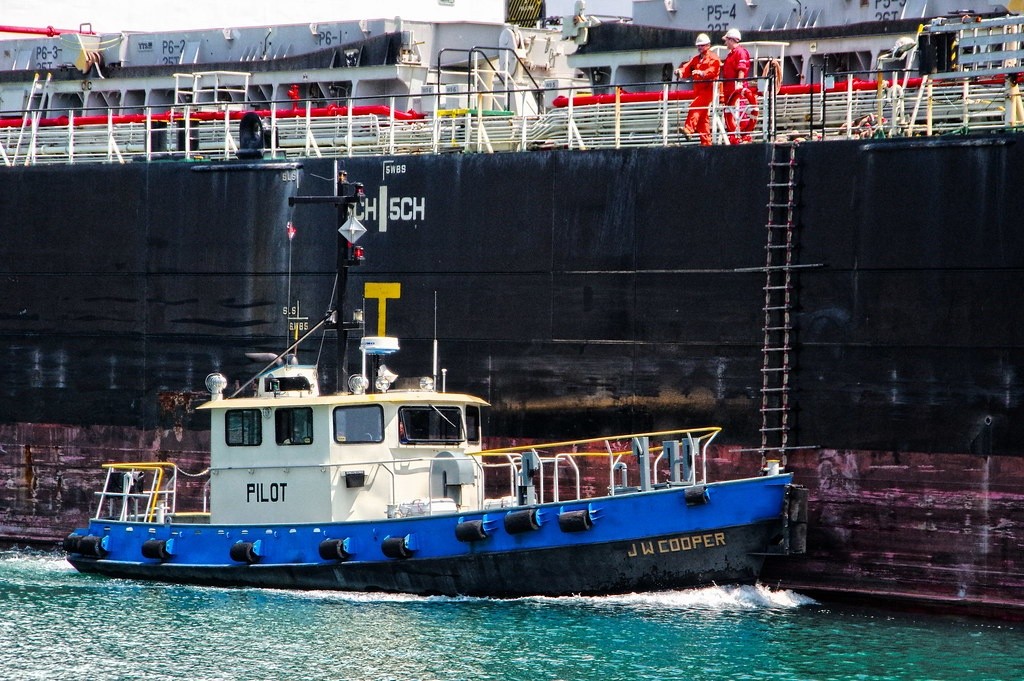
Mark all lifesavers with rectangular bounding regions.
[724,87,759,136]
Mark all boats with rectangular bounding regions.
[65,362,794,604]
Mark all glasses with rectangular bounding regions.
[697,45,705,47]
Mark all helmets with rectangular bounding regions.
[722,29,741,41]
[695,33,711,46]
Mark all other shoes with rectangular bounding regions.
[680,126,692,141]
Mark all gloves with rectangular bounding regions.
[674,69,683,78]
[692,70,702,76]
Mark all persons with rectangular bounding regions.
[674,33,721,146]
[720,28,752,145]
